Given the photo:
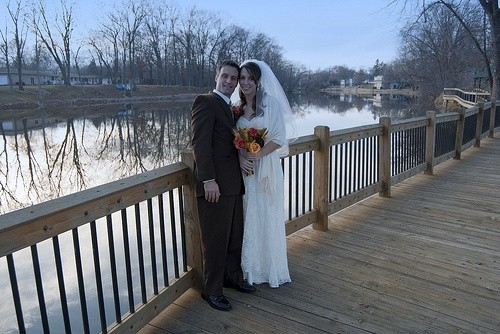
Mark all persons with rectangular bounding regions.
[192,60,258,309]
[230,59,293,289]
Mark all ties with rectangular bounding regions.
[228,100,236,123]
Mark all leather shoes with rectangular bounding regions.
[200,291,233,311]
[235,279,257,293]
